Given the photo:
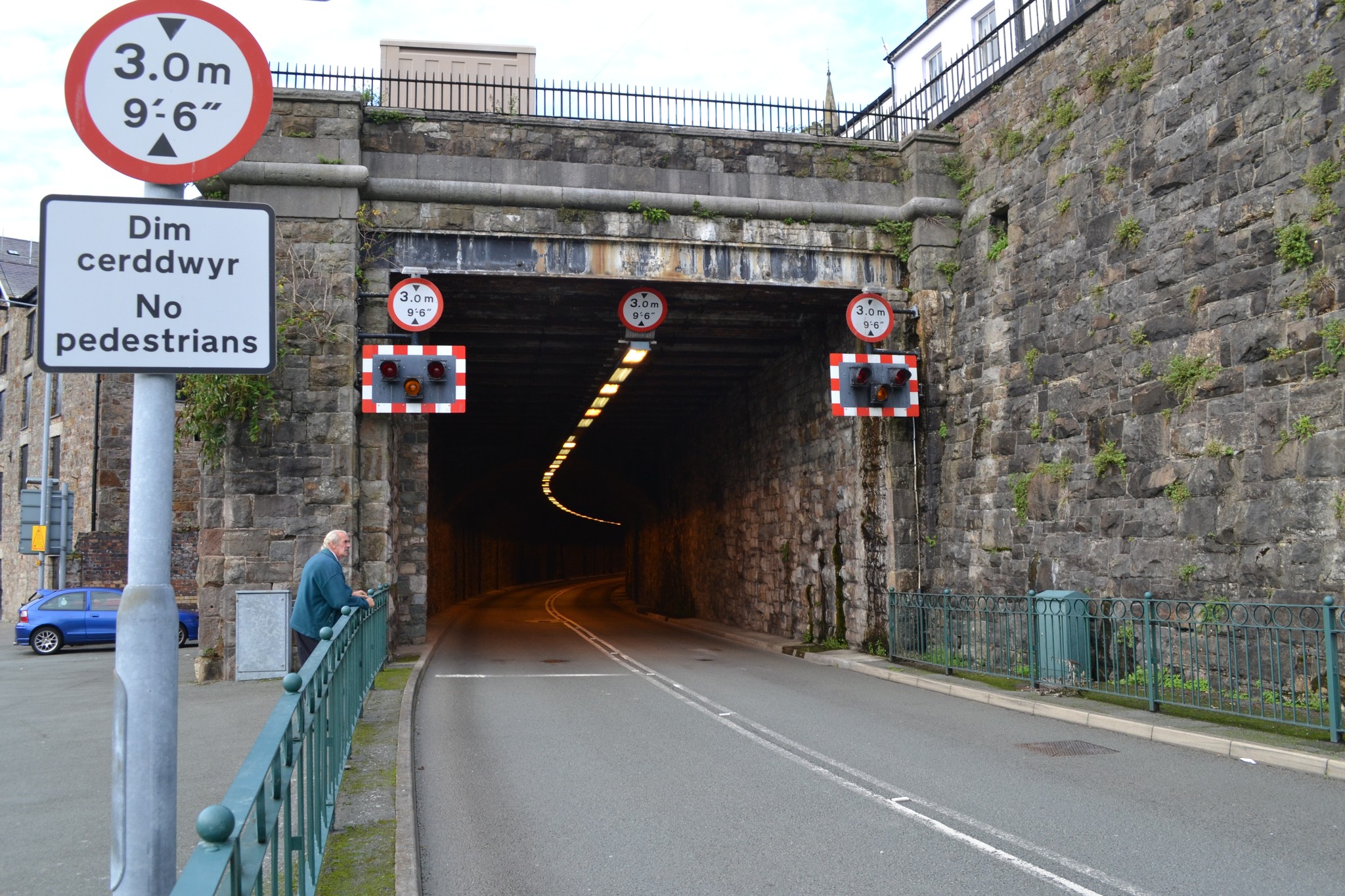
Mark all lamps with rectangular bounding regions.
[619,341,651,364]
[606,361,635,384]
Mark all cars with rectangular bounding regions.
[14,587,199,656]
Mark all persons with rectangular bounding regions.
[289,530,374,733]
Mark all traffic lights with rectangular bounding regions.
[369,355,458,405]
[838,362,911,408]
